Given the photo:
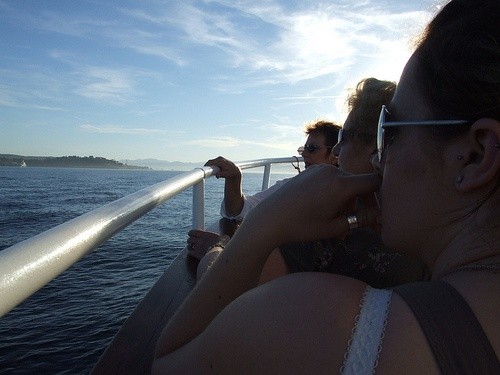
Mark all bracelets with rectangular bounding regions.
[204,245,225,256]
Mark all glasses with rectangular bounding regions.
[338,129,367,143]
[297,144,333,155]
[376,105,476,163]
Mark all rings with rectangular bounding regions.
[345,214,359,229]
[188,243,195,250]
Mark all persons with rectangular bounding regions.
[151,0,500,375]
[186,78,432,290]
[204,121,341,221]
